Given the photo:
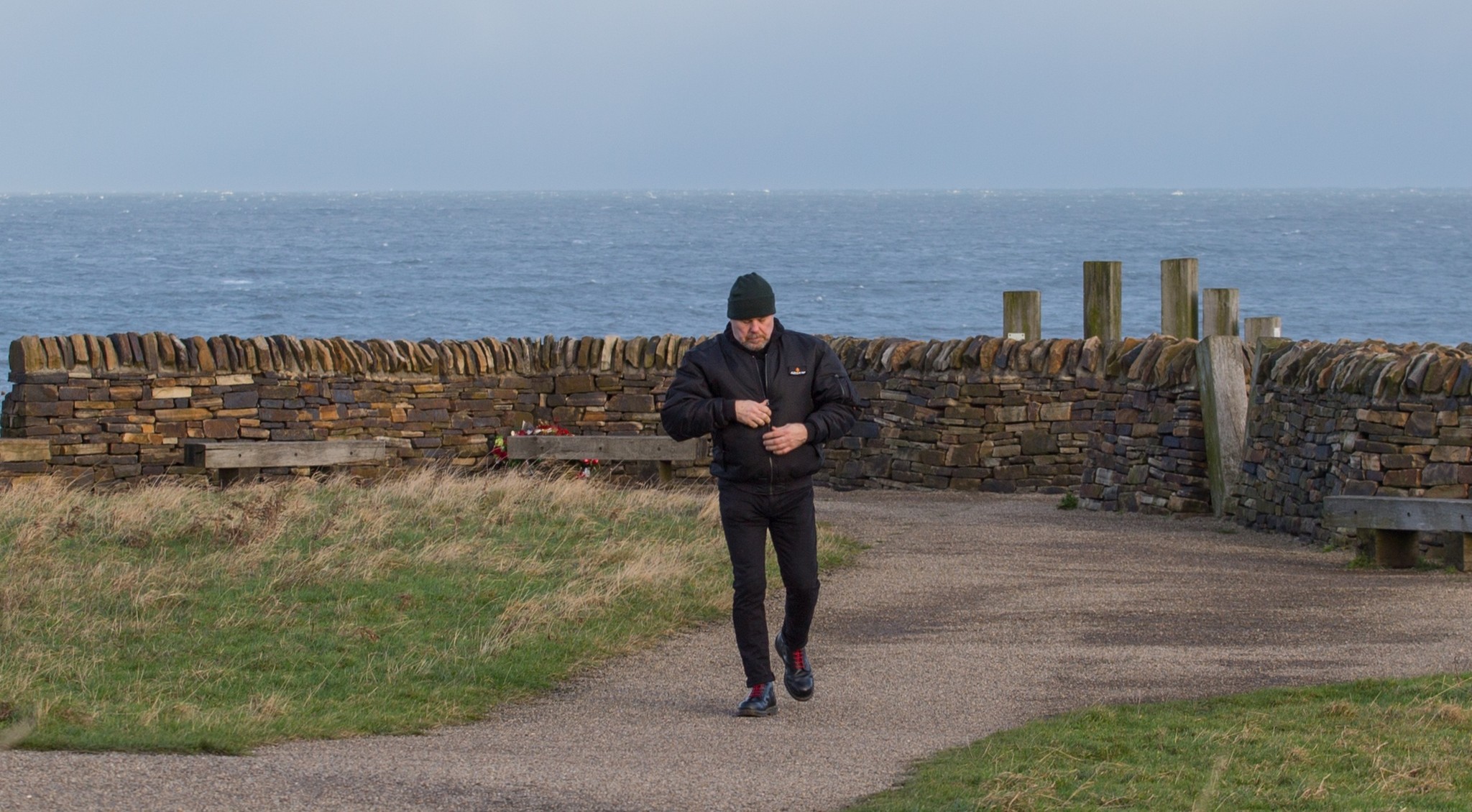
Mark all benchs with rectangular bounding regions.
[1323,494,1472,572]
[505,431,707,487]
[182,440,386,488]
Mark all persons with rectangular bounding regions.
[660,274,863,717]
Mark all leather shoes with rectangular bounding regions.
[774,631,815,701]
[735,681,777,717]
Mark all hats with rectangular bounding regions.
[727,272,776,320]
